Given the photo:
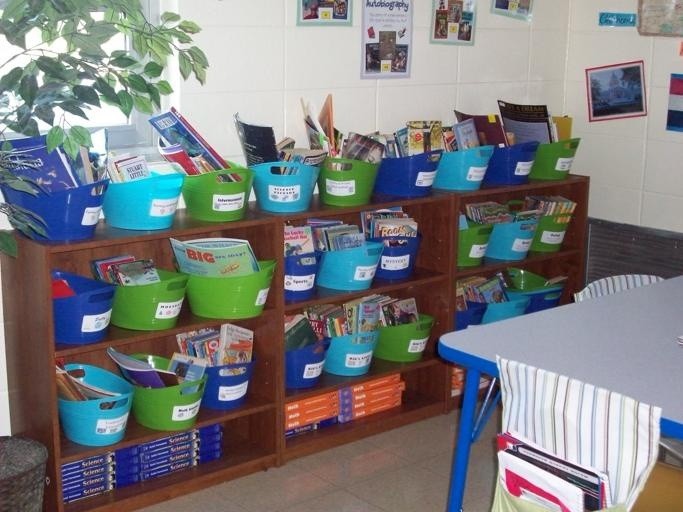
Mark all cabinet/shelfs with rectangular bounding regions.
[3,174,590,512]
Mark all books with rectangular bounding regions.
[56,323,253,409]
[0,133,98,195]
[545,276,566,287]
[495,431,610,512]
[284,294,421,353]
[103,105,243,182]
[456,272,509,311]
[284,206,418,265]
[52,237,259,300]
[233,94,573,174]
[459,195,577,230]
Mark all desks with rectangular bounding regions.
[435,273,682,511]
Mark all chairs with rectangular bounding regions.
[571,274,665,304]
[492,351,666,512]
